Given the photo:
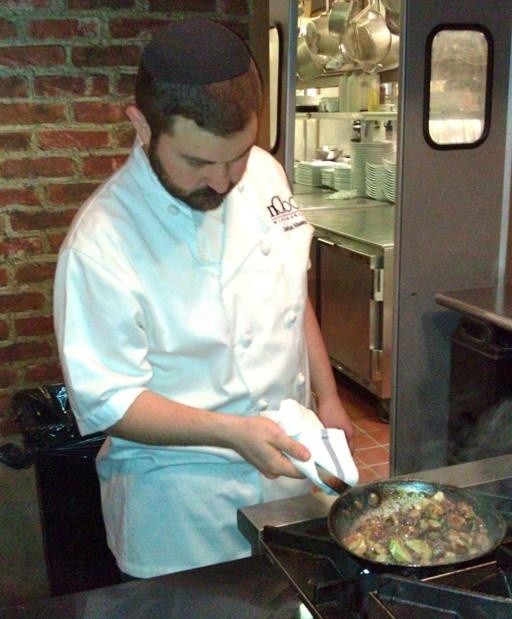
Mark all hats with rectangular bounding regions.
[141,17,252,86]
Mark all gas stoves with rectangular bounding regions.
[260,477,512,619]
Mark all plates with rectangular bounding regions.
[295,161,352,191]
[349,141,397,203]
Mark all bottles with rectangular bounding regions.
[337,70,385,114]
[350,117,393,142]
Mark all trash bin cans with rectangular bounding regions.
[11,384,141,598]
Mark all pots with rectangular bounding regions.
[303,456,507,569]
[294,0,401,83]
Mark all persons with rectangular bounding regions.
[52,7,363,583]
